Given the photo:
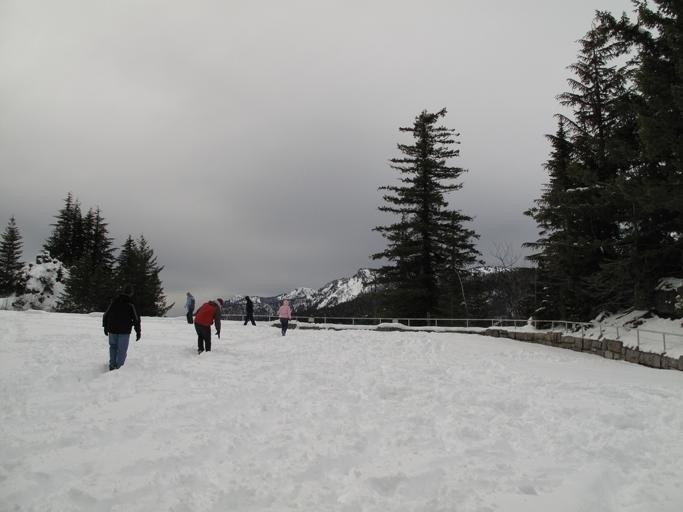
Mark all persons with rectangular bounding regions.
[183,292,195,325]
[101,282,140,370]
[276,299,291,337]
[243,296,256,326]
[192,299,223,352]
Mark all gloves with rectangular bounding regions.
[103,325,111,337]
[134,331,142,341]
[214,331,222,340]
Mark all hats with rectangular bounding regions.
[217,297,224,306]
[122,282,134,295]
